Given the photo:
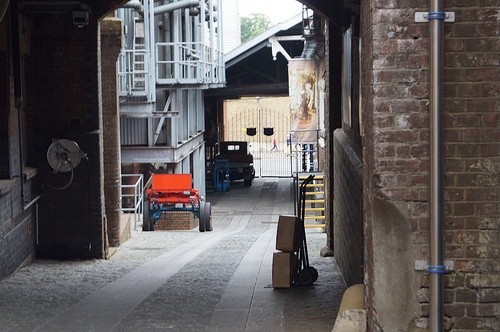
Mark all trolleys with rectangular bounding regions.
[289,174,318,287]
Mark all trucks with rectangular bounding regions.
[214,140,256,188]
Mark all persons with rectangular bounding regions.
[271,135,279,152]
[300,83,313,122]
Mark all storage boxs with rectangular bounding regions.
[275,214,303,252]
[271,252,296,287]
